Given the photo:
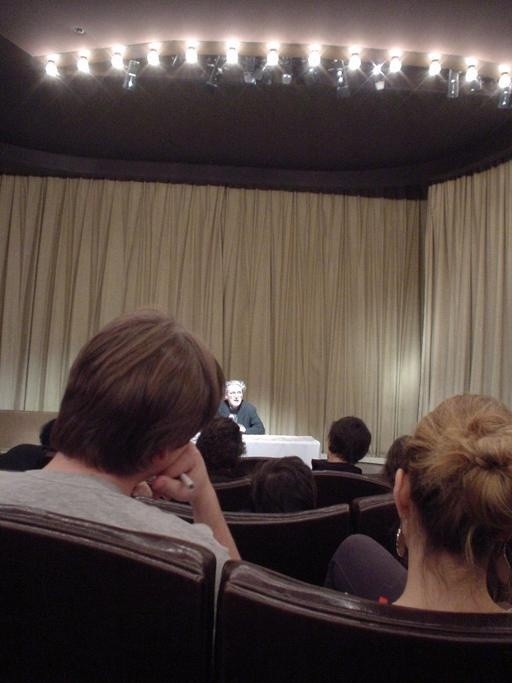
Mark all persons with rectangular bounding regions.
[195,416,246,484]
[323,393,511,615]
[383,434,413,491]
[39,418,56,446]
[318,416,371,475]
[252,455,318,514]
[0,310,242,668]
[217,380,265,434]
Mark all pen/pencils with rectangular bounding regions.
[180,472,194,489]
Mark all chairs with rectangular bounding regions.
[1,457,512,682]
